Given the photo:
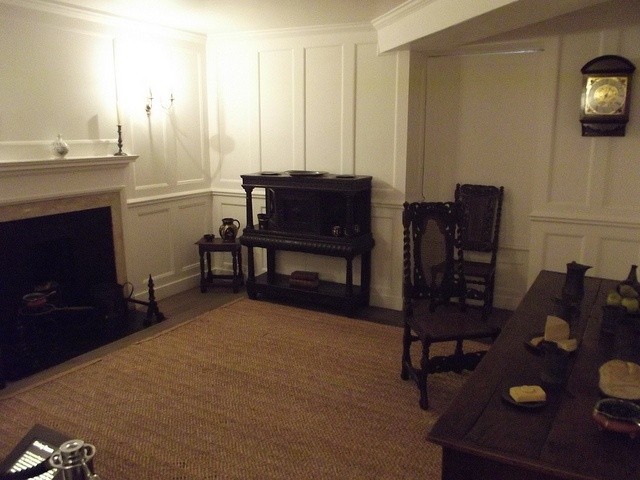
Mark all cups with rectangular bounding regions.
[328,225,343,238]
[599,304,627,337]
[203,234,214,241]
[344,222,361,235]
[257,214,272,230]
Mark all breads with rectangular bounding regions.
[598,359,640,400]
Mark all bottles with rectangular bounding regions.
[49,439,102,480]
[616,264,640,294]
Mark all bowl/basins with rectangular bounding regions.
[539,367,577,399]
[21,292,49,314]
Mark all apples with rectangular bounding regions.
[621,298,639,313]
[606,289,619,308]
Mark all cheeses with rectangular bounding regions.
[508,385,546,402]
[529,316,578,352]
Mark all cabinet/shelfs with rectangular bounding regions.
[240,169,374,315]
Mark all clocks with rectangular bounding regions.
[578,54,636,138]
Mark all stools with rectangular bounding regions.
[195,232,239,293]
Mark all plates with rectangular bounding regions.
[500,386,547,408]
[283,170,329,176]
[259,171,282,175]
[334,174,356,178]
[522,332,582,353]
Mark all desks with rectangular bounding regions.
[425,271,640,480]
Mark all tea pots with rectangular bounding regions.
[219,218,240,243]
[562,260,593,301]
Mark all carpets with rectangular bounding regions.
[0,296,488,478]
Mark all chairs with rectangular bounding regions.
[431,182,503,317]
[400,202,501,409]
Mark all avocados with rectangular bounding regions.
[617,282,637,297]
